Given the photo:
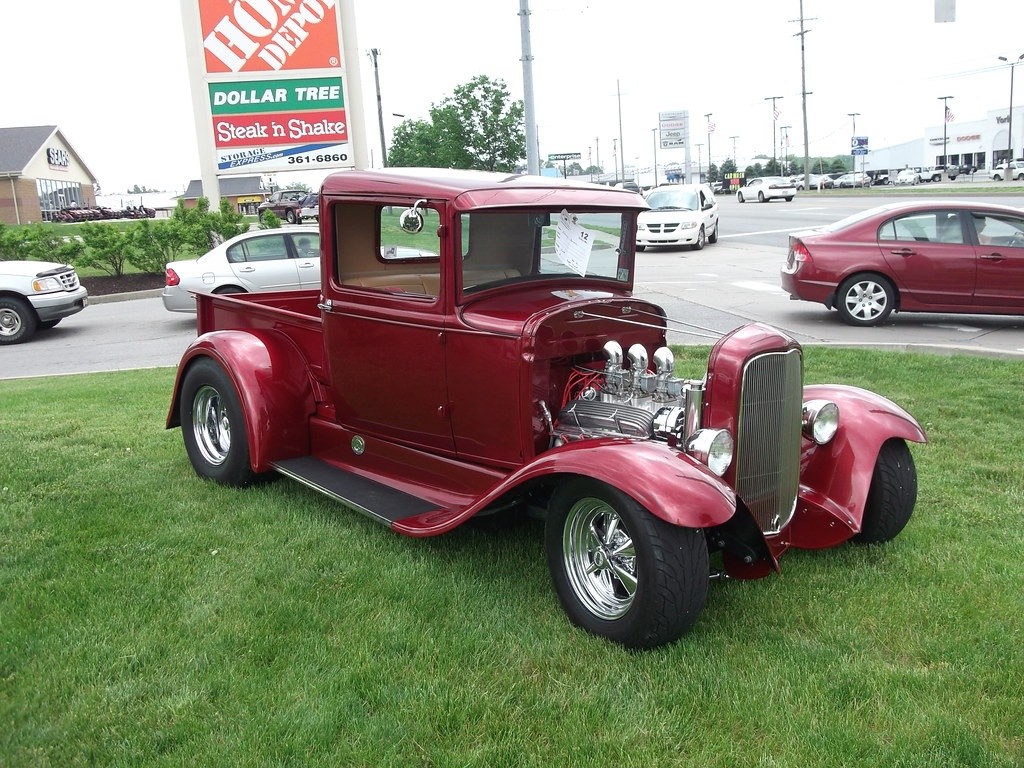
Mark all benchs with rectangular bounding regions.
[341,269,522,297]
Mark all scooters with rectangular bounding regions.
[51,207,156,223]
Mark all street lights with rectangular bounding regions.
[847,112,860,189]
[588,92,793,184]
[999,54,1024,168]
[937,95,954,171]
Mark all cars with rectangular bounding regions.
[989,162,1024,181]
[712,182,724,193]
[166,167,929,649]
[0,259,89,346]
[635,183,719,252]
[735,176,797,203]
[957,164,977,174]
[780,199,1024,327]
[162,228,441,314]
[791,171,892,190]
[893,170,920,186]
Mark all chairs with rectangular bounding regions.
[936,216,963,244]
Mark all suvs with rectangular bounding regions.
[932,164,960,181]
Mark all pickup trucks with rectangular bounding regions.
[914,167,944,183]
[258,190,305,224]
